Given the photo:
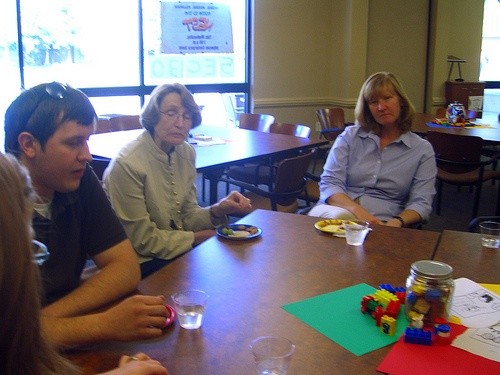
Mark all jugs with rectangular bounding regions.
[447,101,466,123]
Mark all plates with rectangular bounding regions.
[163,303,176,327]
[215,223,263,240]
[314,220,364,234]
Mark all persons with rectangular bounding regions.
[102,83,252,277]
[5,82,170,348]
[310,72,438,228]
[0,152,169,375]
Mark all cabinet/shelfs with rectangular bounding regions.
[445,81,485,118]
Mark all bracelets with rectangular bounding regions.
[393,216,404,227]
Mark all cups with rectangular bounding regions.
[342,219,370,246]
[248,335,295,375]
[172,289,209,329]
[478,221,500,248]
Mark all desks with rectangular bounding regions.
[57,209,500,375]
[411,114,500,147]
[88,122,329,204]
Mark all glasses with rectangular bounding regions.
[32,240,50,266]
[160,109,194,123]
[14,81,67,150]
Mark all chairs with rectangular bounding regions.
[224,108,356,214]
[425,130,500,235]
[96,116,143,133]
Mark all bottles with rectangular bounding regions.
[405,260,456,334]
